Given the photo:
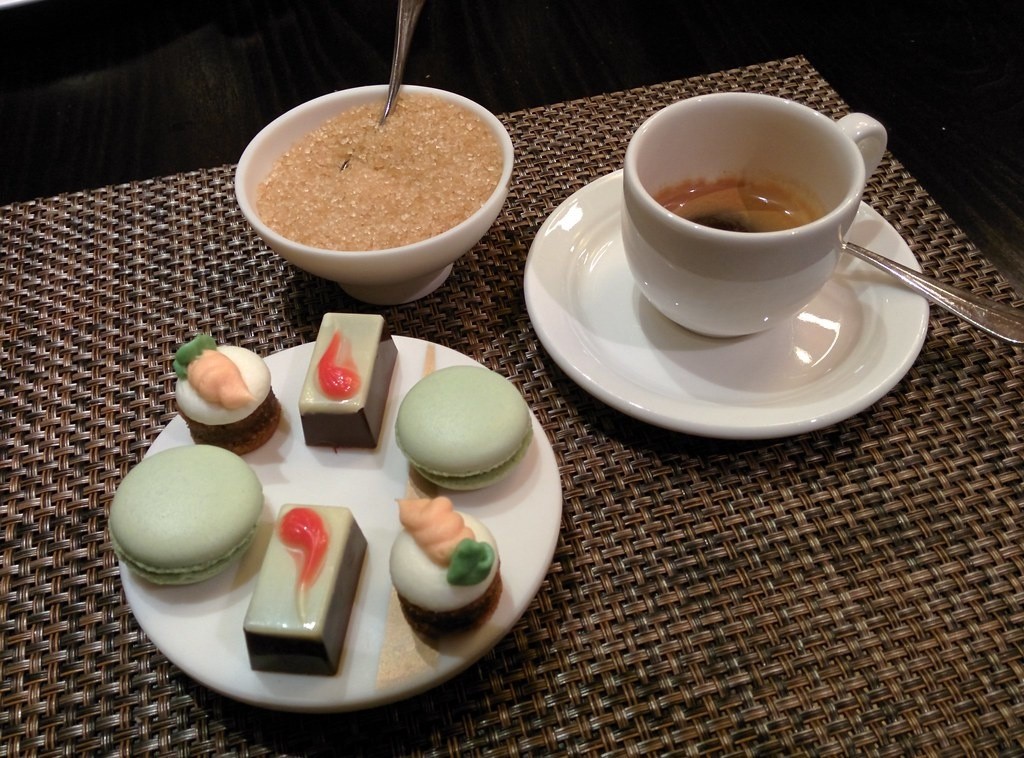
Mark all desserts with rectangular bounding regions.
[299,312,398,449]
[107,442,264,586]
[172,335,282,455]
[394,364,535,491]
[243,503,369,677]
[388,495,503,638]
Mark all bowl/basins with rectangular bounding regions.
[234,84,515,305]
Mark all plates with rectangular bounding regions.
[119,337,564,714]
[524,167,929,441]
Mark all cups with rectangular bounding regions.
[621,91,887,339]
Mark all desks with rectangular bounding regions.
[0,57,1024,758]
